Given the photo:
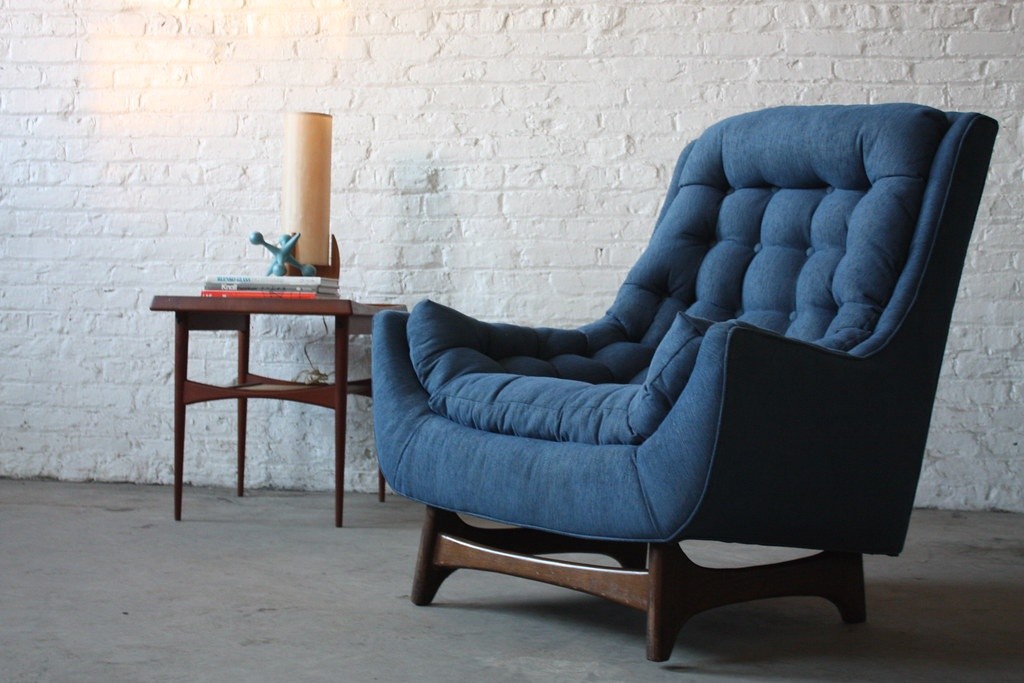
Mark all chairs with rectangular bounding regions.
[372,101,999,662]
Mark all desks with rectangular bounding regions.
[149,295,408,528]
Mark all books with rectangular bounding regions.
[198,274,341,301]
